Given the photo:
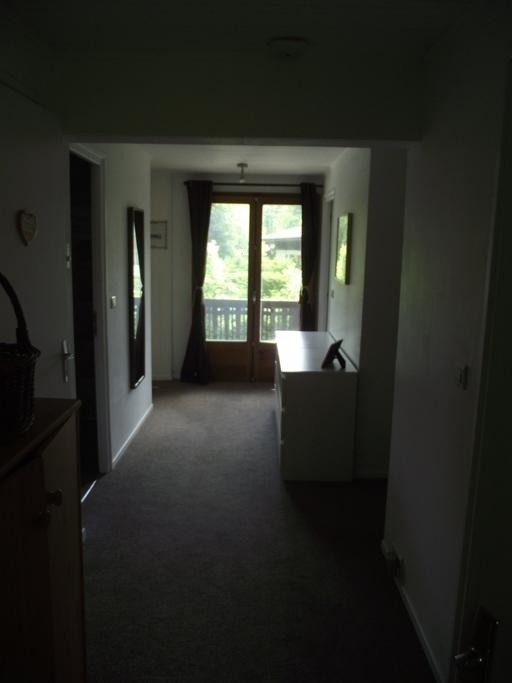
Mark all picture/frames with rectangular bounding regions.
[150,217,169,252]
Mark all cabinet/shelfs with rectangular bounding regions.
[0,396,91,683]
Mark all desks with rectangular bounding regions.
[268,327,358,483]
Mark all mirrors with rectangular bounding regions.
[126,205,147,390]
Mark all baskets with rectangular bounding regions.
[1,276,40,436]
